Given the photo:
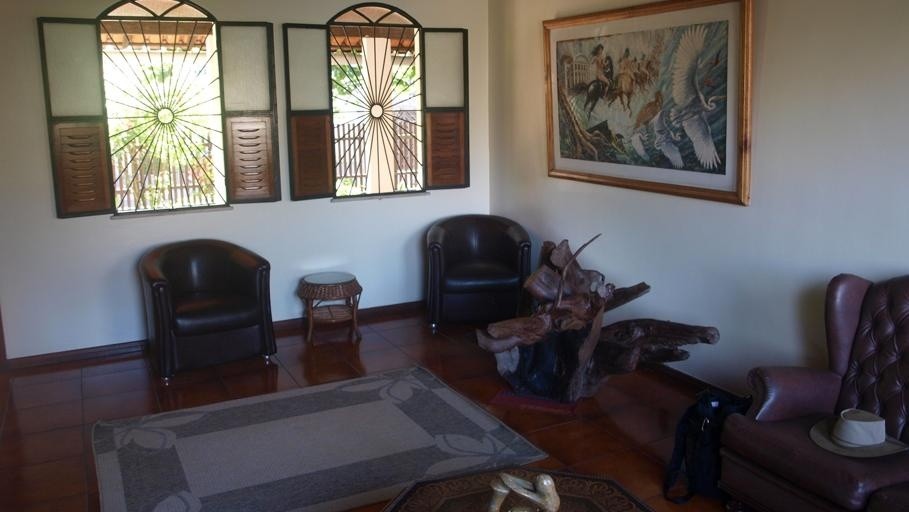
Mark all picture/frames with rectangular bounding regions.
[541,0,753,207]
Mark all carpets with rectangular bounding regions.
[89,365,548,512]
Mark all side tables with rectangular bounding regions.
[297,271,363,346]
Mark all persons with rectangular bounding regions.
[589,43,611,104]
[639,53,653,84]
[616,47,638,96]
[631,55,640,75]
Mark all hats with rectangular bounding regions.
[806,406,909,460]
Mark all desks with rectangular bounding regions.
[378,466,657,512]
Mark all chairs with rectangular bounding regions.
[137,239,277,388]
[718,273,909,512]
[425,214,532,337]
